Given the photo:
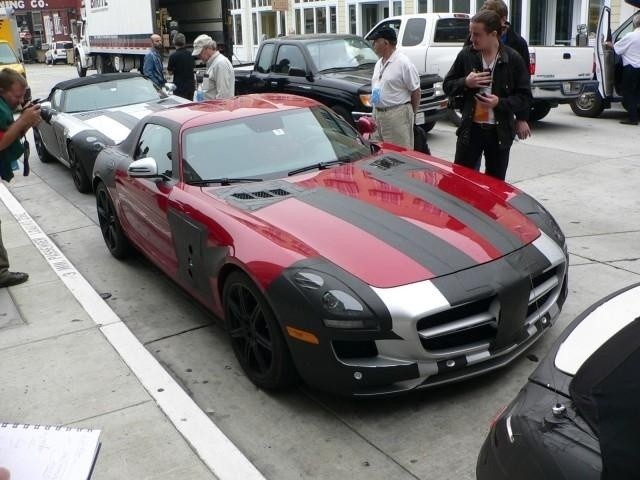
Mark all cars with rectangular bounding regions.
[1,41,27,81]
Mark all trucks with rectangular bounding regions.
[70,0,228,77]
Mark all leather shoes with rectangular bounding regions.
[0,272,29,290]
[620,118,636,125]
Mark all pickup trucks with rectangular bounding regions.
[567,5,640,117]
[365,13,594,127]
[234,34,448,131]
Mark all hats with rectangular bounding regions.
[366,26,397,41]
[192,35,212,56]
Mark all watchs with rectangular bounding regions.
[606,42,608,47]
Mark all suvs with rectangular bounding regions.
[45,41,72,65]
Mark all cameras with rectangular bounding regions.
[30,98,57,126]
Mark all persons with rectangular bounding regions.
[143,33,168,90]
[167,33,195,101]
[1,68,40,286]
[190,33,234,101]
[477,0,532,87]
[602,12,640,127]
[364,26,422,151]
[444,10,532,182]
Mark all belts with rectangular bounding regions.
[471,121,498,130]
[374,101,413,112]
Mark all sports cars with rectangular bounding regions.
[477,279,639,479]
[34,73,192,193]
[92,94,568,393]
